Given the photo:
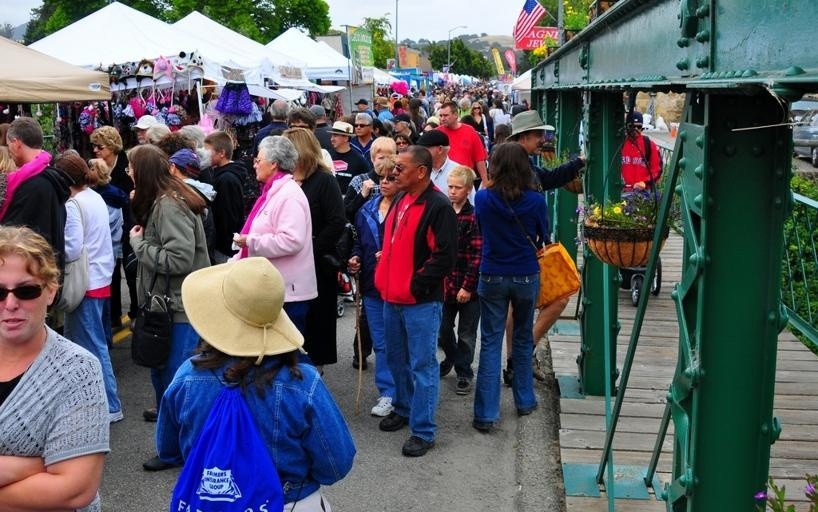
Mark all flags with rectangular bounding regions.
[512,0,547,46]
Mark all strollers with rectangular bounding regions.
[614,183,666,311]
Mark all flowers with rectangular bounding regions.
[570,190,679,244]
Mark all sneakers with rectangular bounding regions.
[109,410,123,423]
[144,407,158,423]
[144,455,170,470]
[353,345,545,457]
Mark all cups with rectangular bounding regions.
[670,125,676,137]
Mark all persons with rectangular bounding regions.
[623,110,663,193]
[0,78,585,473]
[153,255,357,512]
[0,223,112,512]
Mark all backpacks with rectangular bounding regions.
[169,352,308,512]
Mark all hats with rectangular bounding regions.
[326,96,556,146]
[627,111,643,123]
[181,256,308,365]
[94,52,205,173]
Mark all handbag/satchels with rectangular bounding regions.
[536,240,581,309]
[55,247,89,313]
[131,307,172,371]
[334,224,357,270]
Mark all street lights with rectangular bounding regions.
[442,21,468,82]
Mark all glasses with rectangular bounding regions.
[628,124,642,129]
[0,284,48,301]
[378,174,395,181]
[393,165,402,172]
[253,158,274,164]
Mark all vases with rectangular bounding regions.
[583,225,672,268]
